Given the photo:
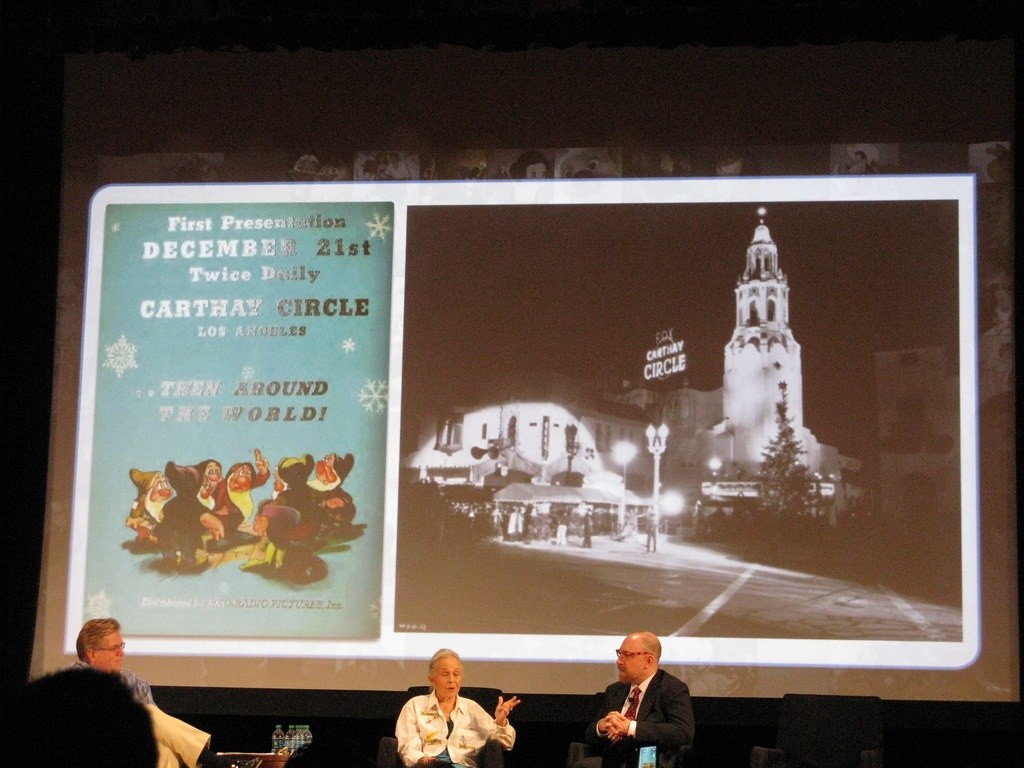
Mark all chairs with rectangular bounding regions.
[565,690,697,768]
[375,686,506,768]
[749,694,886,768]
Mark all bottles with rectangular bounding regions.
[286,725,312,755]
[271,725,285,753]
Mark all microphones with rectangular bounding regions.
[629,697,634,705]
[448,723,452,728]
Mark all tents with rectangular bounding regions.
[494,482,649,509]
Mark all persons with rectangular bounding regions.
[582,631,694,768]
[0,617,263,768]
[395,649,521,768]
[493,505,657,553]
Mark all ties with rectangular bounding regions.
[624,687,641,721]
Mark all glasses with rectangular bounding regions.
[93,642,125,652]
[616,649,654,660]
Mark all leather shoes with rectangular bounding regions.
[202,755,263,768]
